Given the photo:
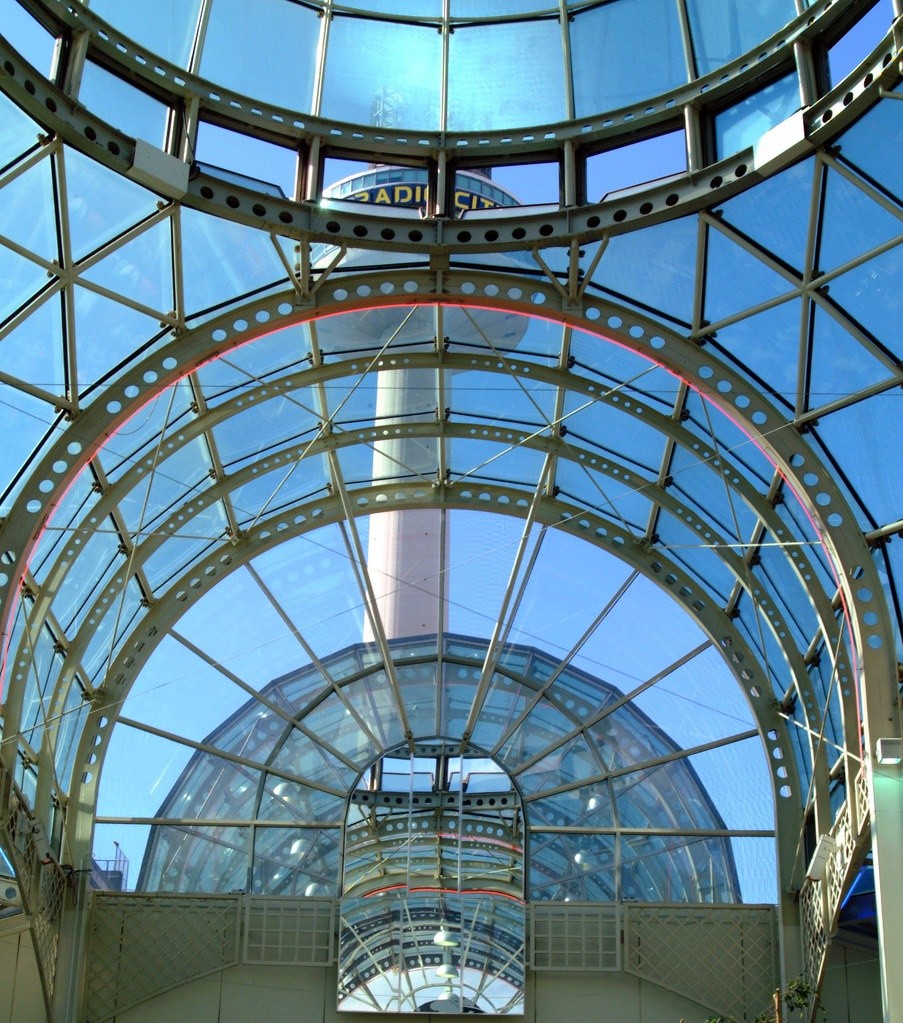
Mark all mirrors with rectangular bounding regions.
[335,735,526,1015]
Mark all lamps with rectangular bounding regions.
[438,978,459,1002]
[434,911,459,947]
[574,849,588,864]
[289,838,315,861]
[434,947,460,978]
[304,882,326,896]
[271,781,301,807]
[587,794,603,811]
[875,737,902,764]
